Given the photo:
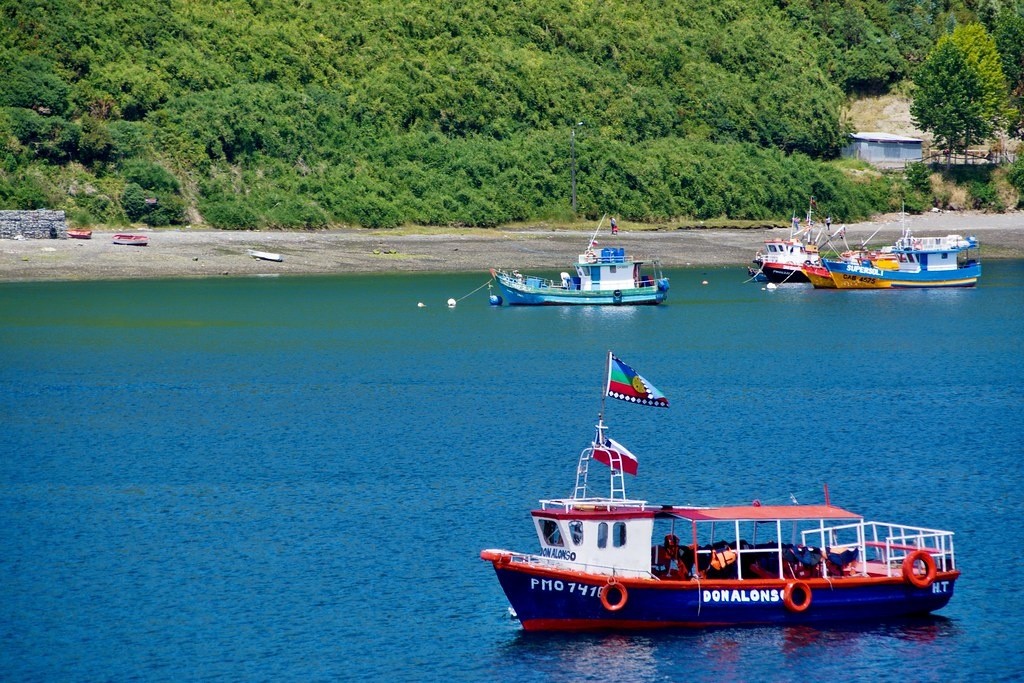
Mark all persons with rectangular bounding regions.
[794,216,799,230]
[610,216,615,234]
[513,270,519,278]
[825,216,830,230]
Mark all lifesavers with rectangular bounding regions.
[782,579,813,612]
[805,260,811,265]
[913,240,923,250]
[613,289,622,299]
[585,252,598,264]
[903,552,938,589]
[599,582,629,612]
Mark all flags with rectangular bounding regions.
[605,351,670,408]
[589,429,638,476]
[811,196,816,208]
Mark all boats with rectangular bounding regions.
[112,234,151,245]
[485,210,671,307]
[817,234,983,290]
[751,196,849,283]
[67,228,93,240]
[250,250,284,263]
[477,351,963,630]
[801,244,902,289]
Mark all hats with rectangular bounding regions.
[664,534,679,547]
